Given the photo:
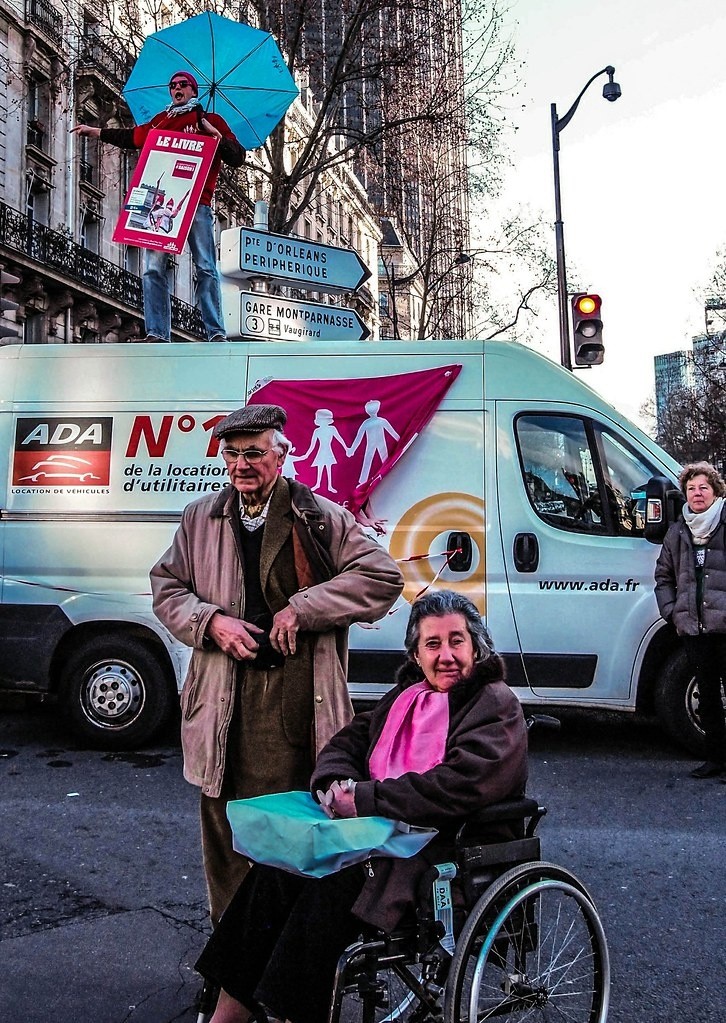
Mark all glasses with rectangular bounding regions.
[169,80,192,88]
[221,446,271,463]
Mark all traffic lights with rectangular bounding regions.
[570,291,605,366]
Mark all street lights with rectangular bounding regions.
[547,65,622,372]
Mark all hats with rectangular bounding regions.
[169,71,198,98]
[214,403,286,440]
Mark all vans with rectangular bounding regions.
[2,341,726,760]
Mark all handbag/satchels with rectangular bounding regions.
[226,790,437,879]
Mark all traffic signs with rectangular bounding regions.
[220,226,372,297]
[220,289,371,343]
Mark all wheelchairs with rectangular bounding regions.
[192,714,612,1022]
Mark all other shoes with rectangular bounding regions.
[689,760,726,778]
[133,336,168,343]
[211,336,228,342]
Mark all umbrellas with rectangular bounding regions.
[121,9,301,150]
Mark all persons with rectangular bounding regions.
[145,194,182,235]
[69,72,246,343]
[655,462,726,784]
[148,403,405,932]
[195,590,530,1023]
